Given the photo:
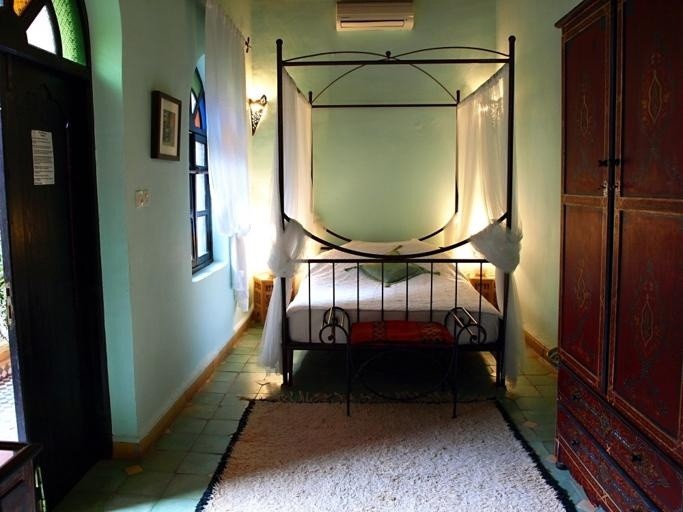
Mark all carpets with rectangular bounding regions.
[196,393,574,512]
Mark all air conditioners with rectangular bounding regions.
[334,1,414,33]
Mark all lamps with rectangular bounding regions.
[248,93,268,136]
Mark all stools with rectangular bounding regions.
[346,321,458,421]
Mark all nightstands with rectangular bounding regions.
[468,273,498,308]
[253,274,296,324]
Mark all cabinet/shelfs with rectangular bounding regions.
[554,1,683,510]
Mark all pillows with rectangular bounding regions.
[344,244,440,288]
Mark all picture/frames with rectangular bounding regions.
[150,89,183,159]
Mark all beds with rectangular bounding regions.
[275,214,511,397]
